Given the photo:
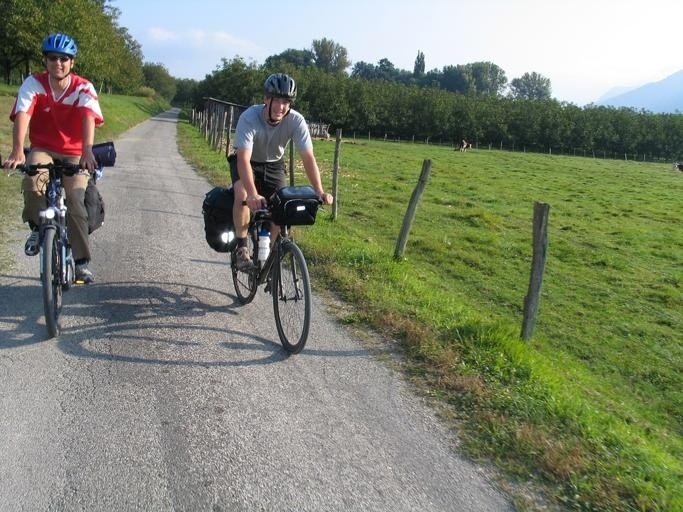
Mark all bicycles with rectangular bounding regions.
[1,163,96,339]
[220,196,323,355]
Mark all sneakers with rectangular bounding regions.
[236,243,258,273]
[25,227,42,256]
[76,264,96,282]
[279,287,285,296]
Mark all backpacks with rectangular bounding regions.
[84,182,105,235]
[202,185,237,252]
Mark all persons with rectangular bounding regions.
[226,70,334,300]
[1,33,105,285]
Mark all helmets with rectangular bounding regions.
[264,73,298,103]
[42,33,77,58]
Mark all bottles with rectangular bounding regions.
[254,228,270,264]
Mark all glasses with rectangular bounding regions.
[46,56,70,62]
